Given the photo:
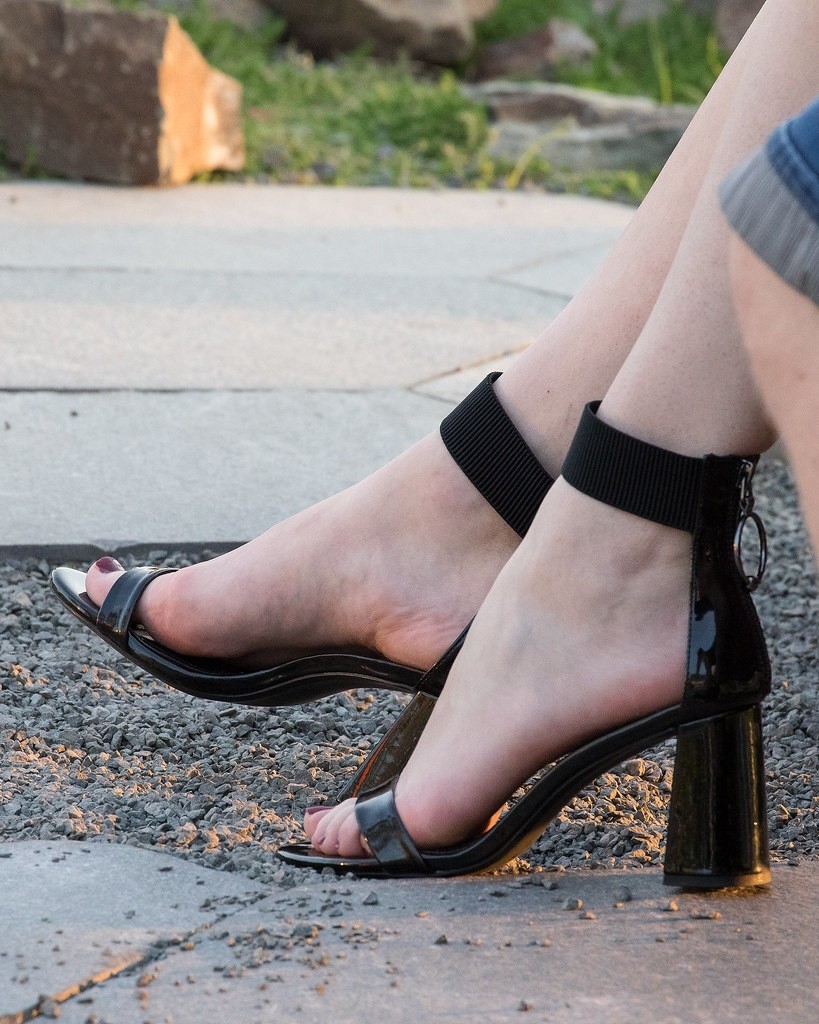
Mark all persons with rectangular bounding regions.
[49,0,819,892]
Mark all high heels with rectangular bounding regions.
[47,370,563,805]
[277,402,772,894]
